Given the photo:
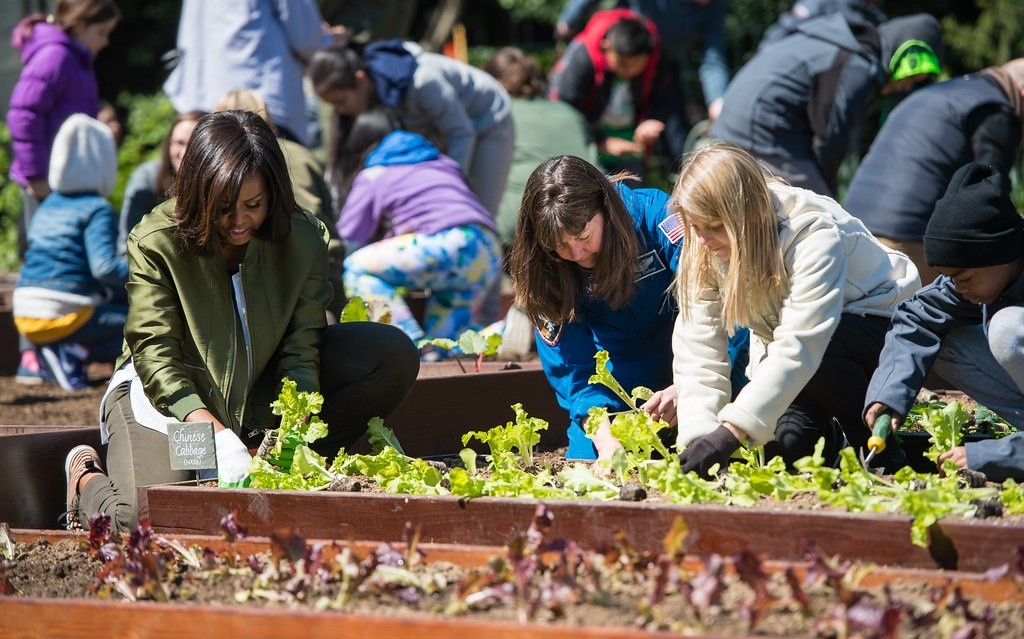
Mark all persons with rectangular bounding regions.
[216,89,347,314]
[548,9,682,187]
[508,152,750,460]
[668,144,924,480]
[161,0,349,143]
[64,111,421,533]
[11,112,130,389]
[483,46,603,274]
[91,99,211,258]
[310,39,517,216]
[760,0,886,202]
[861,162,1024,485]
[334,105,531,363]
[8,0,118,260]
[843,57,1024,285]
[556,0,730,159]
[713,13,943,197]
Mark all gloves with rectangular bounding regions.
[678,425,747,483]
[198,426,253,484]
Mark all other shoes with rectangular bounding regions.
[497,301,534,363]
[417,343,441,363]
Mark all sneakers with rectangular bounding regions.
[57,444,105,532]
[42,341,88,392]
[15,364,49,386]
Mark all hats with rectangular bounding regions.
[925,162,1024,268]
